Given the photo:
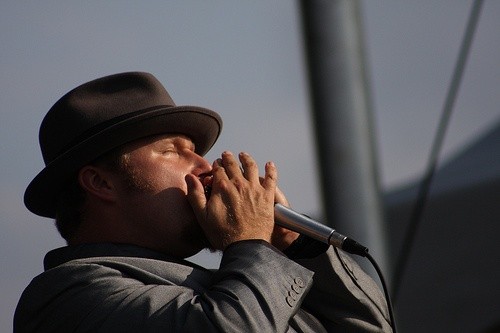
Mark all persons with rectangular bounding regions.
[12,72,393,333]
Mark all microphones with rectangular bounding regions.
[205,178,369,261]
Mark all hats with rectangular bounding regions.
[22,70,223,222]
